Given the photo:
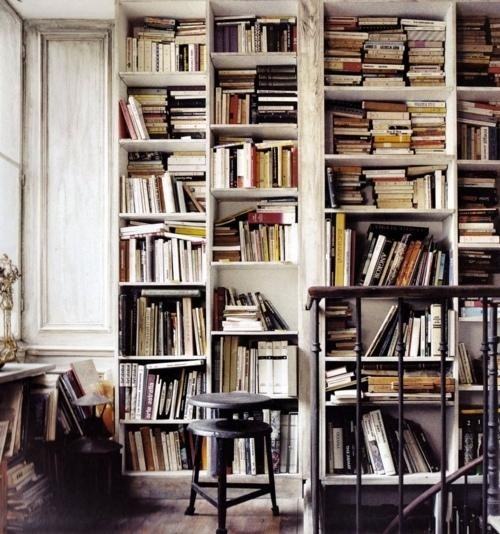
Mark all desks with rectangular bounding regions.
[1,362,58,533]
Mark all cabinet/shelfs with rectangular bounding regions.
[114,0,499,497]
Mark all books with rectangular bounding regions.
[456,15,500,87]
[324,15,446,87]
[325,299,456,356]
[214,14,297,53]
[457,99,500,160]
[214,65,298,125]
[129,425,208,471]
[119,86,206,141]
[0,379,57,464]
[120,151,206,213]
[324,501,479,534]
[118,287,207,356]
[56,359,104,438]
[326,408,441,475]
[213,197,299,263]
[326,165,448,210]
[210,136,298,188]
[458,250,493,286]
[458,295,499,317]
[325,212,452,286]
[120,217,206,283]
[459,169,500,244]
[119,360,207,421]
[213,287,289,331]
[125,16,208,72]
[457,341,500,387]
[6,460,55,533]
[212,336,298,397]
[325,101,447,155]
[458,403,500,475]
[231,409,298,474]
[326,362,455,401]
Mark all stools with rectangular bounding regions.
[185,394,278,533]
